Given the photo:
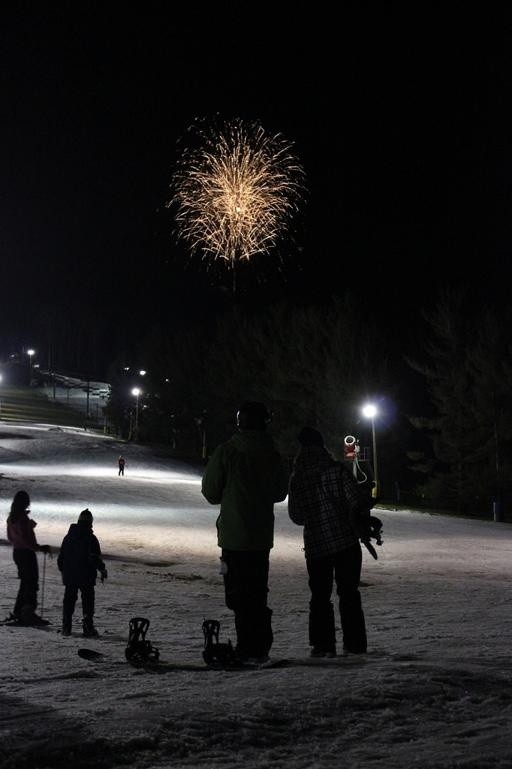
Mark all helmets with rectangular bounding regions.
[236,403,274,428]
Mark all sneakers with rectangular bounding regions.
[311,646,336,658]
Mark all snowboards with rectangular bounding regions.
[77,617,287,670]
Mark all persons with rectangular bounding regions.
[117,454,126,475]
[200,403,286,664]
[287,425,370,659]
[57,507,111,636]
[6,489,52,620]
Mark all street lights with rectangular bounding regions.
[359,401,381,498]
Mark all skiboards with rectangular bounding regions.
[0,617,53,627]
[56,626,104,640]
